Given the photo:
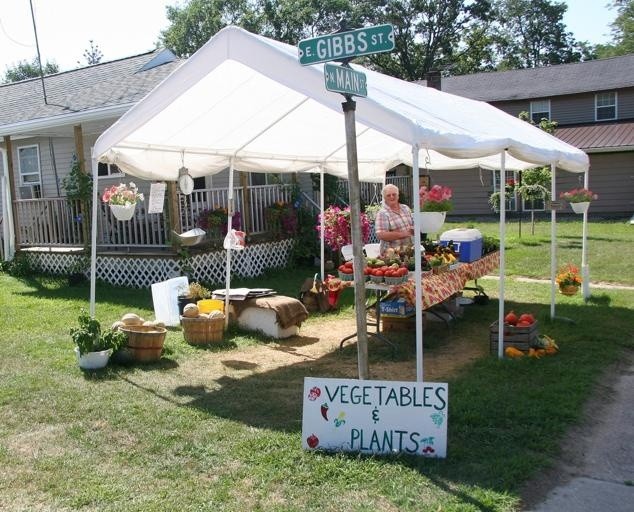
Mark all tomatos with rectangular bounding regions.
[506,313,534,327]
[339,262,353,274]
[364,266,408,277]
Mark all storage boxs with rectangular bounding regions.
[440,228,483,263]
[490,318,537,360]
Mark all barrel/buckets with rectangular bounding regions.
[117,324,167,362]
[179,314,226,346]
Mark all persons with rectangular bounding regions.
[374,183,413,255]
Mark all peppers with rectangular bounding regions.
[321,402,329,421]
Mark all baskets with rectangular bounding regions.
[558,264,577,295]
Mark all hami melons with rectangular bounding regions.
[112,313,165,331]
[184,303,224,319]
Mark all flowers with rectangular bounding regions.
[560,188,598,202]
[103,181,145,206]
[315,204,370,252]
[266,200,298,233]
[197,204,241,236]
[557,265,582,287]
[420,184,454,211]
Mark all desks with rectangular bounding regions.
[332,249,500,360]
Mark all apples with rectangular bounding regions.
[307,432,319,448]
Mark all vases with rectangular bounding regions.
[420,211,447,234]
[111,204,138,221]
[570,202,590,214]
[560,285,577,296]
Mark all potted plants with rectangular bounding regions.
[69,307,127,371]
